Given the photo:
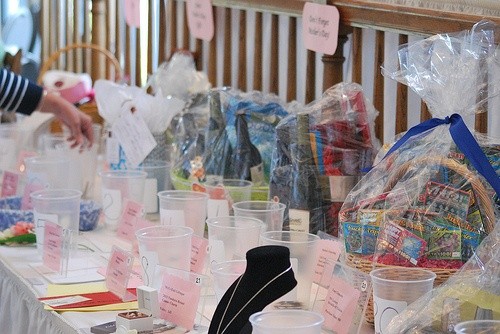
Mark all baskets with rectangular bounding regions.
[339,156,496,331]
[35,44,121,152]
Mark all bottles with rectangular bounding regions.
[181,93,326,235]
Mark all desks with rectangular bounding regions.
[0,215,373,334]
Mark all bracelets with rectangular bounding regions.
[36,88,48,112]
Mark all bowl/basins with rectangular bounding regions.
[0,195,98,230]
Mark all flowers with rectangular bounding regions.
[0,222,95,252]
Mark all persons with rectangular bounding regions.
[0,0,42,84]
[0,65,94,153]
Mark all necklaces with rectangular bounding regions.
[217,264,292,334]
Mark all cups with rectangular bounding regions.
[157,190,209,254]
[454,320,500,334]
[24,123,101,209]
[249,310,324,334]
[370,267,437,334]
[261,231,321,311]
[30,187,82,261]
[134,223,194,291]
[99,171,147,234]
[205,216,263,279]
[129,159,171,221]
[204,178,254,217]
[212,259,248,304]
[232,200,286,241]
